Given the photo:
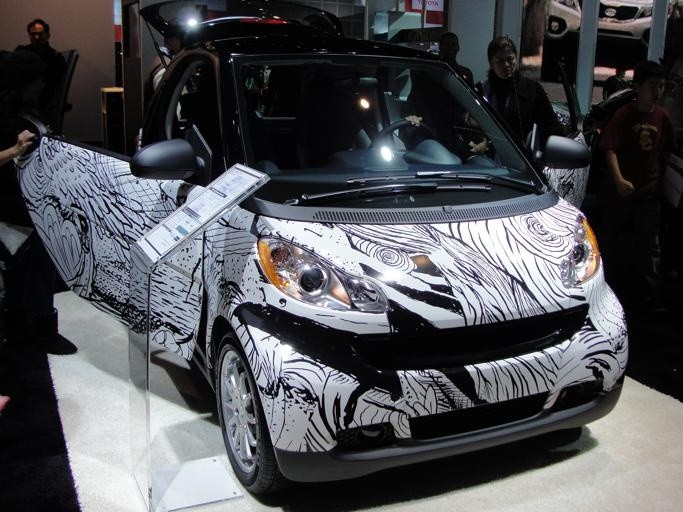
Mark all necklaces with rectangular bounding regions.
[490,77,525,149]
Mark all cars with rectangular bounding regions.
[11,3,631,504]
[544,0,675,60]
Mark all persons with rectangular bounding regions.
[397,116,422,151]
[407,30,474,118]
[475,34,564,158]
[6,19,66,128]
[601,66,628,100]
[593,59,676,310]
[140,31,195,139]
[452,119,495,160]
[0,129,78,356]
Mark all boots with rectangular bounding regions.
[31,308,78,354]
